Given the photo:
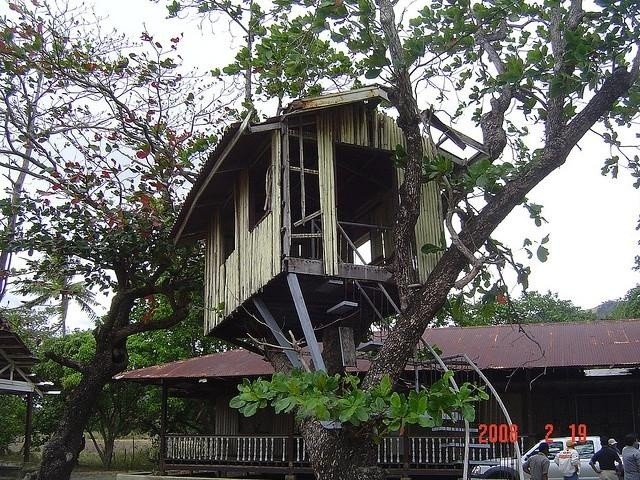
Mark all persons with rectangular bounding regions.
[523,435,639,480]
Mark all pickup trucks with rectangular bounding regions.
[468,434,625,480]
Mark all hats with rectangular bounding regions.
[566,440,573,447]
[607,439,617,446]
[536,443,548,453]
